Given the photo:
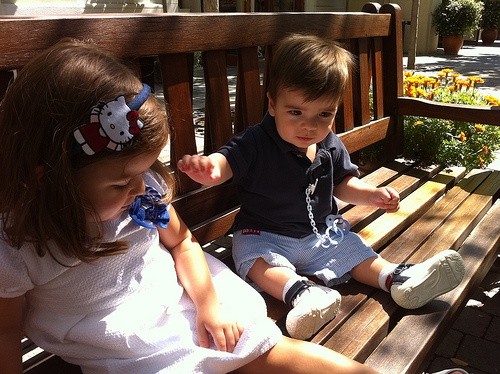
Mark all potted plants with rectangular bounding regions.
[475,0,500,45]
[433,0,484,57]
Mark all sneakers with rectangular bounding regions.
[384,248,466,311]
[285,279,342,341]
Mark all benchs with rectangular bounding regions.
[0,0,500,374]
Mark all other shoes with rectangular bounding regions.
[422,368,468,374]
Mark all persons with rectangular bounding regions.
[0,38,469,374]
[177,33,465,338]
